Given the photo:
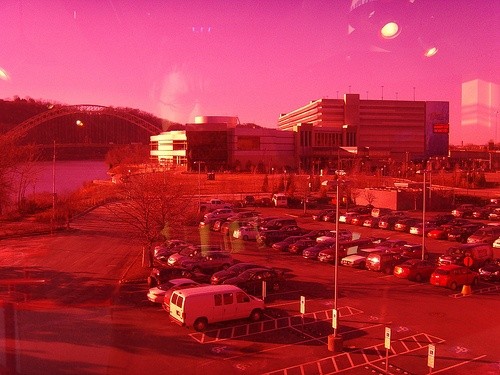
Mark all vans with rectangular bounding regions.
[317,238,369,265]
[179,249,233,273]
[259,219,298,230]
[271,193,288,208]
[169,284,266,332]
[166,244,223,268]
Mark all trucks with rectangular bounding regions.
[436,242,494,269]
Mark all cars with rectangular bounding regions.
[153,239,185,257]
[302,243,331,260]
[398,243,430,260]
[272,235,307,251]
[312,199,500,248]
[203,207,233,221]
[211,262,274,285]
[392,259,437,282]
[232,226,264,241]
[241,196,257,208]
[478,258,500,282]
[340,251,370,269]
[255,229,290,246]
[223,268,281,294]
[155,244,194,263]
[155,242,189,263]
[226,212,259,222]
[288,240,319,254]
[429,263,481,290]
[146,278,201,304]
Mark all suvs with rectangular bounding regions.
[335,169,348,176]
[364,250,406,275]
[162,283,211,316]
[315,229,353,244]
[147,267,210,288]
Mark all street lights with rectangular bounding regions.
[320,179,351,353]
[416,169,429,261]
